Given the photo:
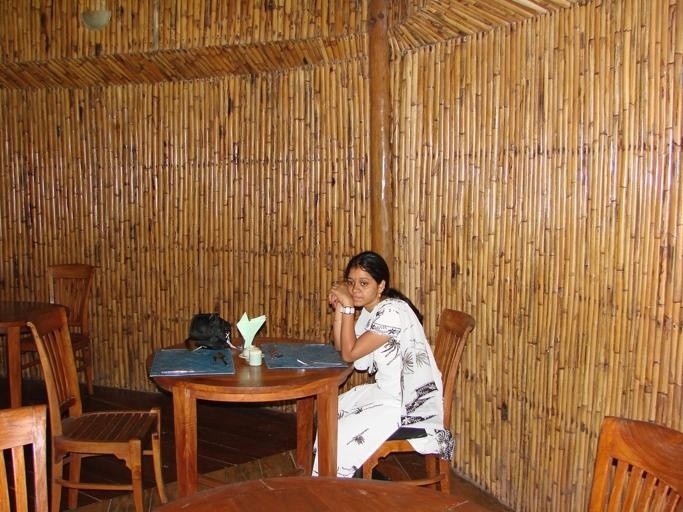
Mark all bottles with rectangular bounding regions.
[239,343,265,361]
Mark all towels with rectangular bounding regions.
[237,311,267,346]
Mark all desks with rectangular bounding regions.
[146,337,355,496]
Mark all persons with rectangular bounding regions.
[310,251,456,479]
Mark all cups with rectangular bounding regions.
[249,349,262,366]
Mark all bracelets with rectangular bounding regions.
[340,306,356,314]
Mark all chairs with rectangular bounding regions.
[21,263,96,395]
[587,417,683,512]
[0,405,50,512]
[362,308,474,493]
[27,306,169,512]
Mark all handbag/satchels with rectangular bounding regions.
[188,313,233,349]
